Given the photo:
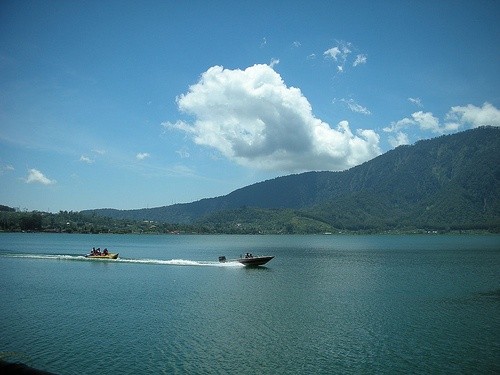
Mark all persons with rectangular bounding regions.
[246,253,253,258]
[96,248,101,256]
[90,248,96,256]
[103,248,109,256]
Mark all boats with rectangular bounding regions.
[236,253,275,268]
[84,247,120,260]
